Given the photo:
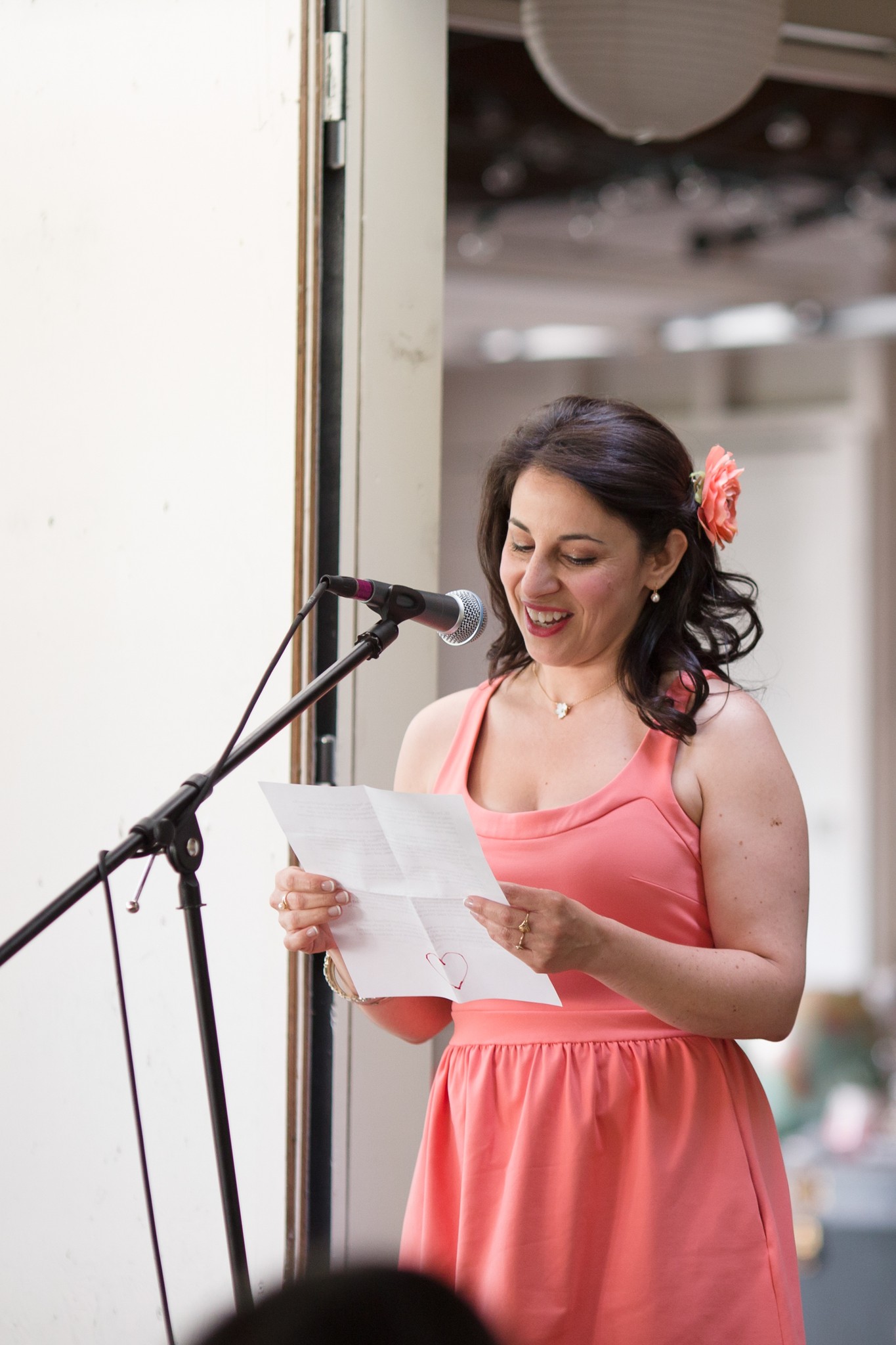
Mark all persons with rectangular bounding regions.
[190,1262,502,1343]
[267,392,812,1343]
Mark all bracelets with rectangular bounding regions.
[321,953,388,1005]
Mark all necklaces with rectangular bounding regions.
[531,661,626,719]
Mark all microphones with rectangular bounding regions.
[318,574,489,648]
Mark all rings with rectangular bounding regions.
[278,890,292,912]
[514,933,527,951]
[519,912,532,932]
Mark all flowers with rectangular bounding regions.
[687,441,738,552]
